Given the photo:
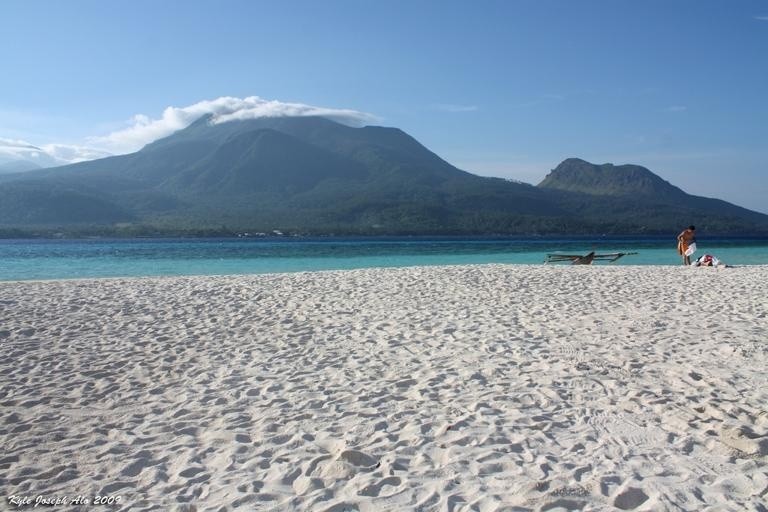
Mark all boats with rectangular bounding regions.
[569,251,594,265]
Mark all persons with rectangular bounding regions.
[676,224,697,267]
